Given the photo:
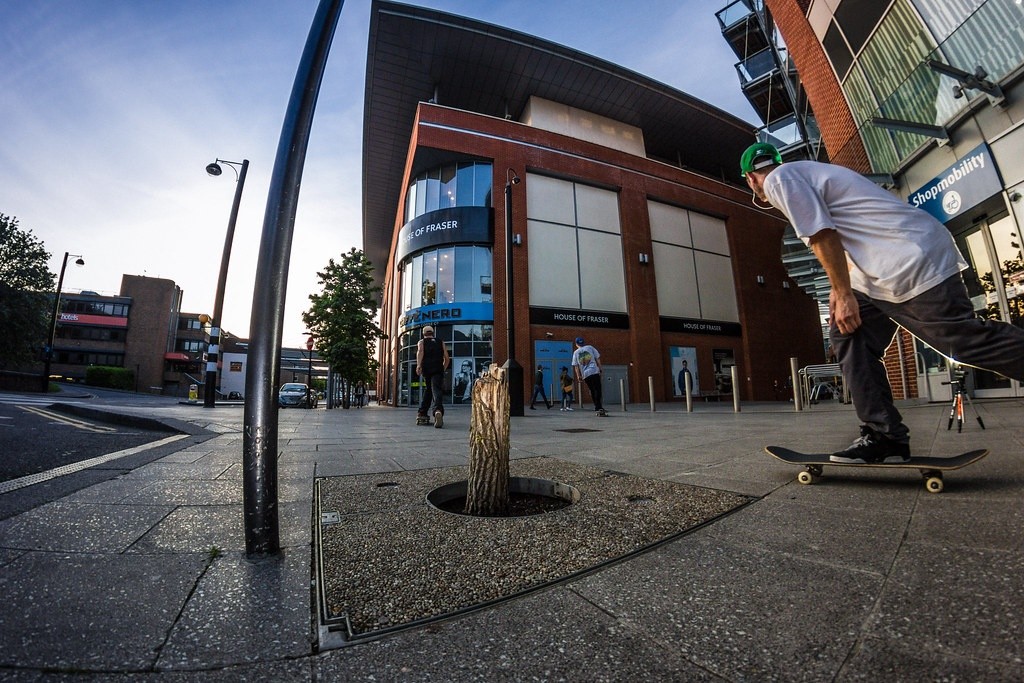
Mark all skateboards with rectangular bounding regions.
[765,445,990,493]
[595,410,608,417]
[415,416,430,425]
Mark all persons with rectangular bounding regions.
[560,366,574,411]
[740,142,1023,466]
[416,325,450,428]
[678,359,693,395]
[355,381,366,409]
[481,360,492,373]
[571,337,609,417]
[454,359,472,404]
[529,364,554,410]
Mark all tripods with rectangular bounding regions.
[942,378,986,433]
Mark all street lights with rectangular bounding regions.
[203,159,249,408]
[39,251,85,396]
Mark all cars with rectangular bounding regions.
[278,382,308,408]
[307,389,318,409]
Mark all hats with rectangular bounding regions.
[576,337,584,344]
[740,143,782,177]
[423,326,433,334]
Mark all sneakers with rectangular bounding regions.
[416,412,430,420]
[434,410,443,428]
[830,426,911,464]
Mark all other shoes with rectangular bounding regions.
[547,405,554,409]
[529,406,536,410]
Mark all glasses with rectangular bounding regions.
[462,365,469,368]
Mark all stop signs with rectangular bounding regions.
[307,337,314,351]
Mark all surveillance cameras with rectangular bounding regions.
[511,176,520,184]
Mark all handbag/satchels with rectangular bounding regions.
[564,384,573,392]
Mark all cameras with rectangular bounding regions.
[953,370,969,378]
